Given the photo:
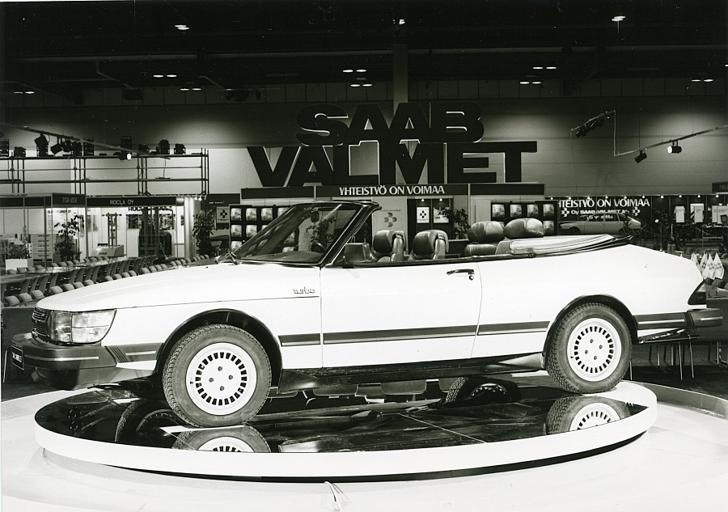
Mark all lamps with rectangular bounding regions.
[634,151,646,163]
[34,130,63,155]
[668,141,681,154]
[117,150,132,161]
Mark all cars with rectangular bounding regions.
[8,199,724,427]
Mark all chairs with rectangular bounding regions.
[371,217,544,261]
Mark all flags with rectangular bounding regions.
[680,249,725,297]
